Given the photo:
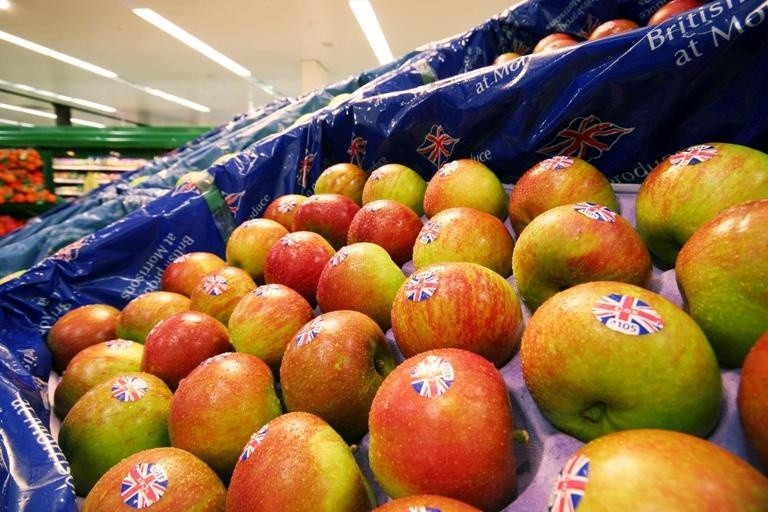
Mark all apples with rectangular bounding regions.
[532,32,575,54]
[44,140,767,511]
[646,1,703,27]
[492,51,520,66]
[588,18,640,42]
[545,39,580,53]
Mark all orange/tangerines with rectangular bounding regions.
[0,147,60,205]
[0,214,27,236]
